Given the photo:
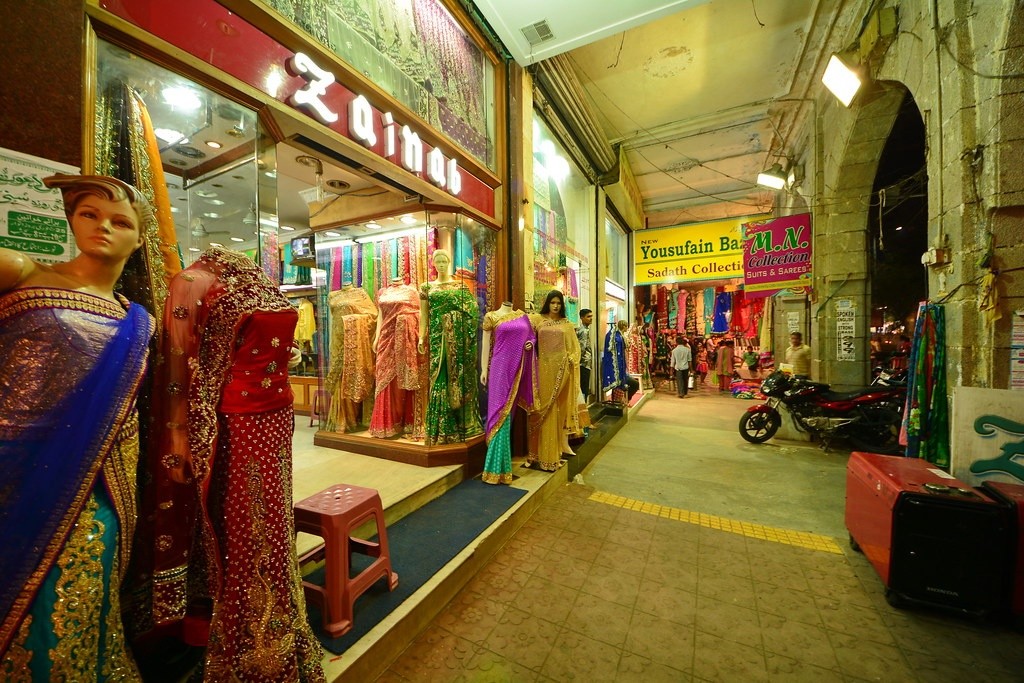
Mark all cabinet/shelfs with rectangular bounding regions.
[282,290,329,417]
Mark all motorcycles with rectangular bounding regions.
[739,349,909,455]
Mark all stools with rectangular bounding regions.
[293,483,399,639]
[310,390,331,427]
[630,374,643,395]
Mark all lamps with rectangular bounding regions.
[820,51,866,110]
[756,163,787,189]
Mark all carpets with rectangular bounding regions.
[607,392,645,408]
[303,478,529,657]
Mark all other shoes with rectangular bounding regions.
[679,394,684,398]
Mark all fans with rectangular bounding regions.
[176,201,257,239]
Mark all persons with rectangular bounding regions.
[481,301,540,485]
[785,332,812,379]
[525,290,582,472]
[870,335,912,356]
[325,249,485,447]
[154,248,327,683]
[576,309,593,405]
[740,346,759,372]
[669,337,736,398]
[0,172,157,683]
[618,320,639,408]
[627,323,653,389]
